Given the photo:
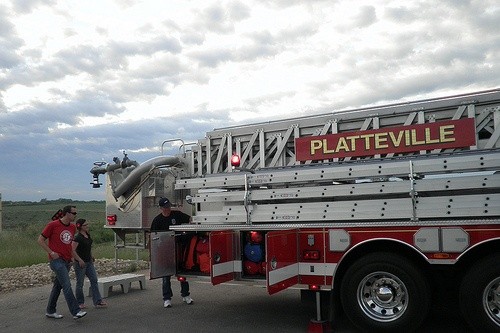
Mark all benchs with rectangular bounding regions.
[88,274,145,298]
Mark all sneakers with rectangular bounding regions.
[183,296,194,304]
[164,299,173,307]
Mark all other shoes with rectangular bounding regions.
[45,313,64,319]
[79,304,86,308]
[73,311,87,320]
[96,302,106,307]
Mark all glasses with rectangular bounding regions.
[69,212,78,215]
[82,223,89,226]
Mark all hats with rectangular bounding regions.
[158,197,172,207]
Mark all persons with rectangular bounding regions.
[71,218,107,309]
[151,198,194,308]
[37,205,87,319]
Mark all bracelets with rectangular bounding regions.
[49,251,54,255]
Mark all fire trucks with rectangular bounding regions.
[91,85,500,333]
